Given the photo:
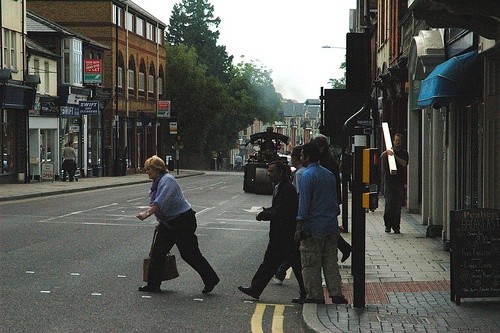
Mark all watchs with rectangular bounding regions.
[145,211,150,217]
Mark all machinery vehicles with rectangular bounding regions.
[242,126,291,195]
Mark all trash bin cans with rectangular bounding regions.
[168,156,174,171]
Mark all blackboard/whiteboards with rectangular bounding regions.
[449,208,500,297]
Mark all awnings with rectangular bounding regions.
[418,50,484,110]
[407,0,500,41]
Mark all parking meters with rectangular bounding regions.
[360,147,381,213]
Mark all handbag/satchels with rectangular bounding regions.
[142,228,180,282]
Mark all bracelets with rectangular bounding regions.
[296,228,303,231]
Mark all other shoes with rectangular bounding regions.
[138,283,162,293]
[272,275,283,285]
[340,245,353,262]
[332,296,349,304]
[292,297,305,303]
[201,279,220,295]
[237,285,259,298]
[300,299,326,305]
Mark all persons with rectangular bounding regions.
[238,134,352,304]
[236,153,242,171]
[216,155,223,171]
[135,156,220,294]
[380,134,409,234]
[61,140,78,182]
